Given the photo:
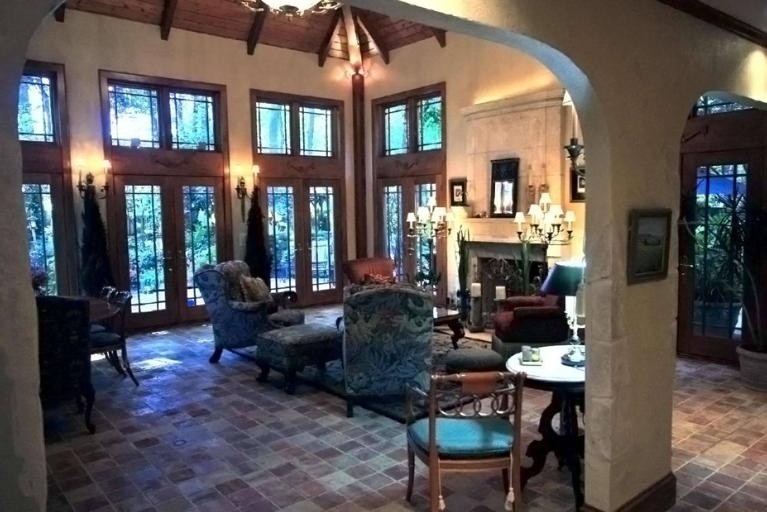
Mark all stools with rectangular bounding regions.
[445,348,504,384]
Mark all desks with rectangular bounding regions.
[52,294,121,323]
[505,345,586,511]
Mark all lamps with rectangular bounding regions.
[239,0,345,19]
[560,89,586,181]
[73,158,112,200]
[513,192,576,287]
[233,164,261,223]
[405,195,452,292]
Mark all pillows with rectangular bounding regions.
[239,273,275,306]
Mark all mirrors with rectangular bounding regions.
[488,158,518,218]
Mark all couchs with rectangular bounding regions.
[340,287,433,418]
[490,263,587,361]
[192,260,304,366]
[255,322,342,394]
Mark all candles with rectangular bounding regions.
[540,166,545,185]
[527,166,532,186]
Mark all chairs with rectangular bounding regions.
[89,290,140,390]
[36,295,98,433]
[83,286,122,374]
[342,255,398,286]
[404,370,526,511]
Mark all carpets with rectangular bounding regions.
[228,314,492,424]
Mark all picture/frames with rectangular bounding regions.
[624,207,670,286]
[568,164,586,203]
[448,179,467,205]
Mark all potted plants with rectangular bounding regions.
[694,191,765,393]
[455,225,470,326]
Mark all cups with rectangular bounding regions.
[521,346,540,362]
